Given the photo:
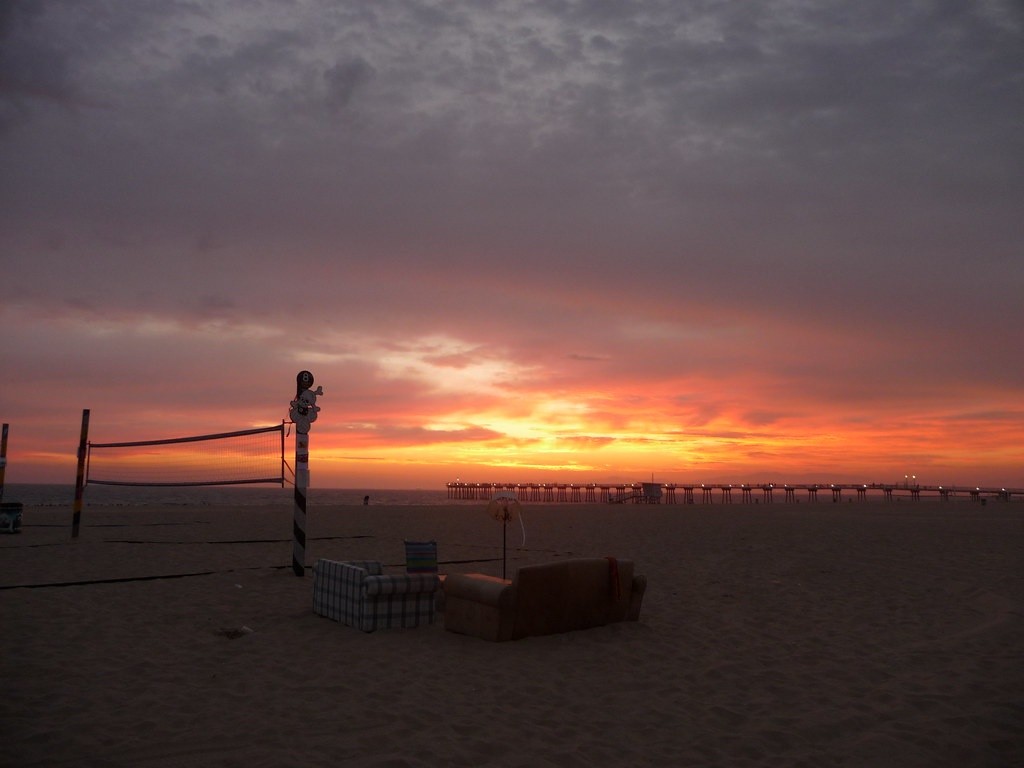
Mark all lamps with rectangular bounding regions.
[487,491,522,580]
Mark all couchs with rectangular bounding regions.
[312,558,442,633]
[441,555,649,643]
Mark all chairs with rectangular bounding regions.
[404,541,438,575]
